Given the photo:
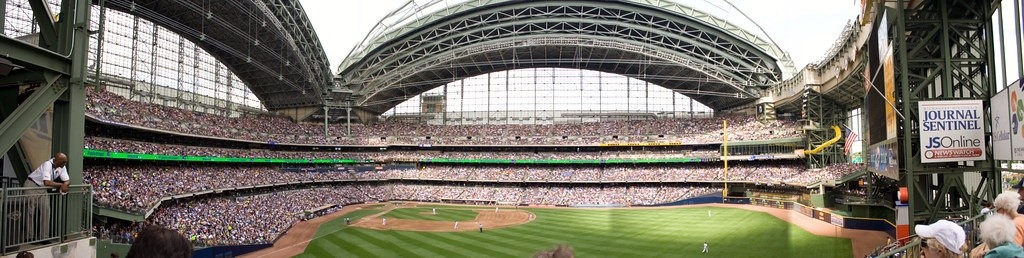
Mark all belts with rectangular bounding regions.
[27,177,41,187]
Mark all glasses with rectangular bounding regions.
[919,238,929,250]
[991,208,1002,213]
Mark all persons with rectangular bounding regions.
[24,152,72,246]
[83,84,901,257]
[968,177,1024,257]
[914,219,969,258]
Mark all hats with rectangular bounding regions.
[914,219,966,255]
[1012,178,1024,188]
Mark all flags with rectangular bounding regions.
[843,127,857,155]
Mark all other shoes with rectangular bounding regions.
[39,240,51,245]
[24,238,38,245]
